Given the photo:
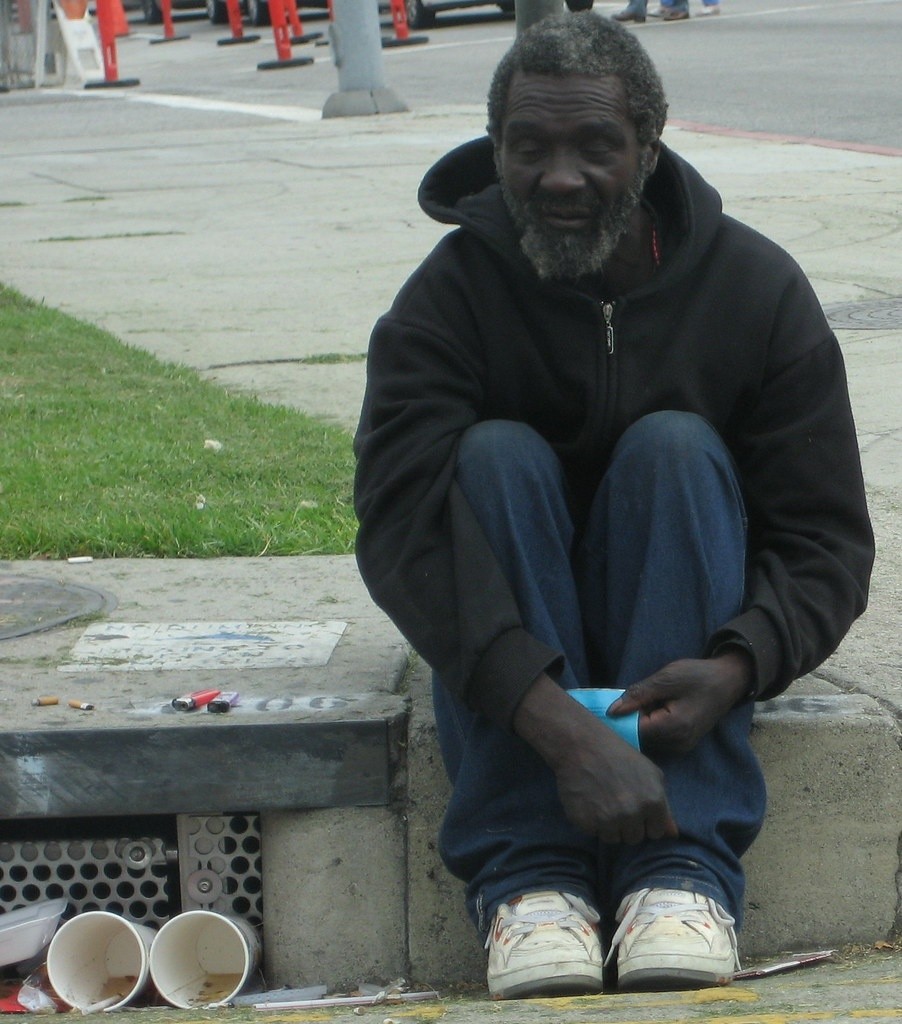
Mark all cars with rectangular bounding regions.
[139,0,594,31]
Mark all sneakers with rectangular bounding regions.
[603,886,742,985]
[484,889,605,998]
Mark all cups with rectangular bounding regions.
[46,911,159,1013]
[148,910,262,1010]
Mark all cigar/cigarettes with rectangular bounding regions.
[31,697,58,705]
[69,699,94,710]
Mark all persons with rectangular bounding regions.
[566,0,721,24]
[354,10,876,1000]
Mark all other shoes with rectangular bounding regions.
[648,8,664,16]
[701,7,720,14]
[664,11,689,20]
[611,5,646,22]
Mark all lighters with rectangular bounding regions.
[172,688,242,712]
